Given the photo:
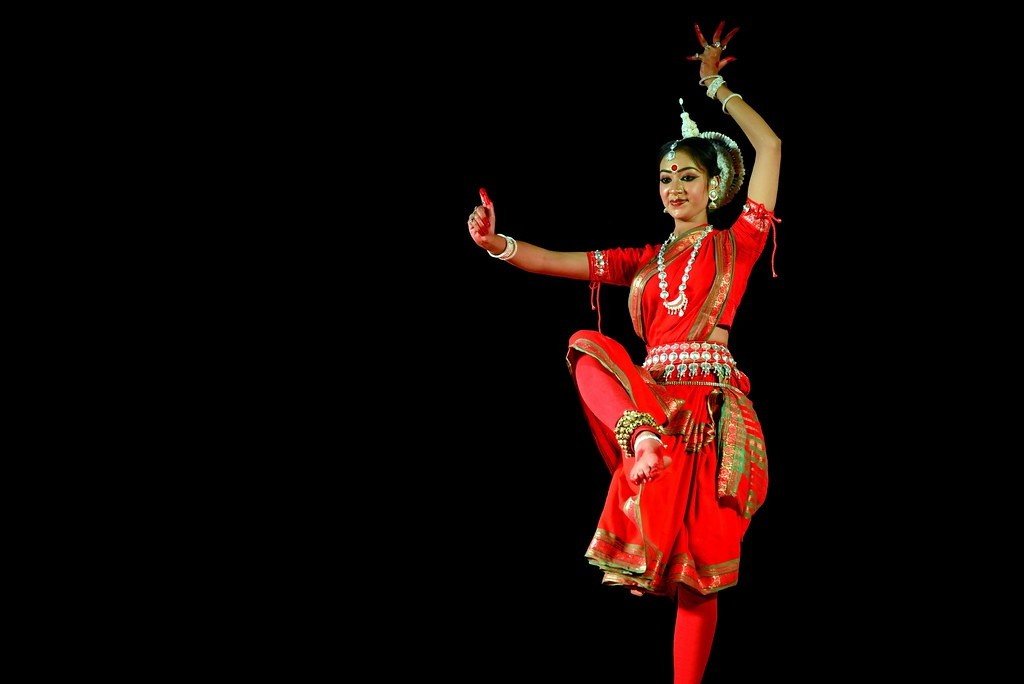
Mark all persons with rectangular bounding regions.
[468,21,782,684]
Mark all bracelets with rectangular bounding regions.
[699,75,723,86]
[707,78,727,99]
[487,234,518,260]
[722,94,743,114]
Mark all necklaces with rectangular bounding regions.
[657,224,713,316]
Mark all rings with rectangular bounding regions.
[473,206,478,215]
[696,53,699,59]
[703,45,712,50]
[713,41,721,48]
[470,217,474,223]
[720,44,727,51]
